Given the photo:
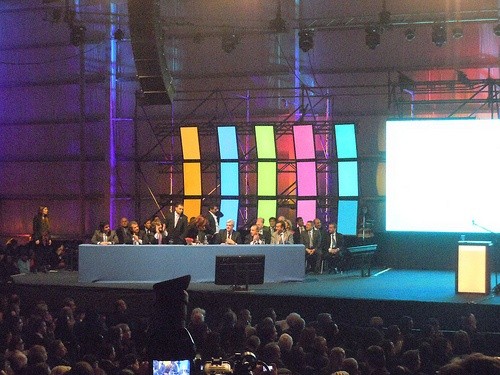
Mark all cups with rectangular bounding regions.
[461,235,465,240]
[261,239,265,244]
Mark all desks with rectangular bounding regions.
[78,244,306,284]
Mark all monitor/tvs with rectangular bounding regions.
[338,244,378,275]
[214,255,266,290]
[149,354,195,375]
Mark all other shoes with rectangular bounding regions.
[310,266,314,271]
[328,268,332,274]
[32,268,38,275]
[334,268,339,274]
[314,267,320,274]
[48,269,58,272]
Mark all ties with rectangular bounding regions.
[228,233,230,239]
[309,231,313,248]
[332,234,336,249]
[279,234,283,244]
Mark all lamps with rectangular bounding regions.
[114,27,124,41]
[298,27,315,51]
[365,28,380,49]
[404,27,415,41]
[431,17,448,47]
[221,31,240,53]
[270,0,287,32]
[451,20,464,39]
[378,0,392,24]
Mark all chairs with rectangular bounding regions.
[321,259,343,274]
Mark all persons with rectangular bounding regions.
[173,360,179,375]
[0,201,500,375]
[157,361,166,375]
[164,364,172,375]
[181,367,190,375]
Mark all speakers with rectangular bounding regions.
[456,240,492,295]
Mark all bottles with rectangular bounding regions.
[204,235,207,245]
